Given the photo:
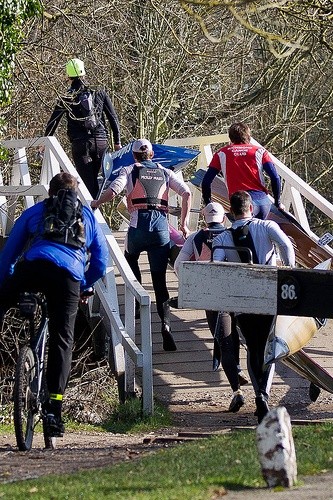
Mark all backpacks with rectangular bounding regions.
[24,198,86,255]
[67,89,98,130]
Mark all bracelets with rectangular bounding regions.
[179,223,186,227]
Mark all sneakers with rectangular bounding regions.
[238,371,248,386]
[43,414,64,434]
[229,394,244,412]
[256,394,270,424]
[160,324,176,351]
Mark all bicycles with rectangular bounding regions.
[6,285,89,451]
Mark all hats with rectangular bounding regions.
[132,139,152,152]
[66,59,85,77]
[202,203,224,223]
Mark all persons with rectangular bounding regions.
[173,200,248,413]
[211,190,295,421]
[201,123,285,221]
[44,59,123,213]
[0,171,110,434]
[89,138,191,352]
